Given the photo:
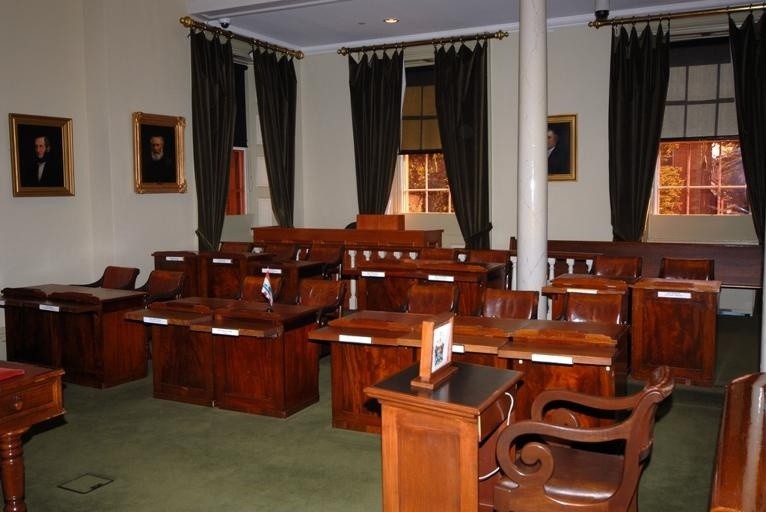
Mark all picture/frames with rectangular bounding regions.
[129,110,188,196]
[545,113,579,184]
[7,111,76,198]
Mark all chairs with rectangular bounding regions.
[494,364,676,511]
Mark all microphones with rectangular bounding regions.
[195,229,216,252]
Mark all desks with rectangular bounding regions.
[362,360,524,511]
[2,207,724,443]
[0,359,66,512]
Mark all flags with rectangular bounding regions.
[262,272,273,305]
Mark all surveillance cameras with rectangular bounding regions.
[218,17,231,28]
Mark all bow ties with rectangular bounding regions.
[35,159,45,164]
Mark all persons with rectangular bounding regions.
[22,137,63,187]
[143,136,176,184]
[545,129,567,174]
